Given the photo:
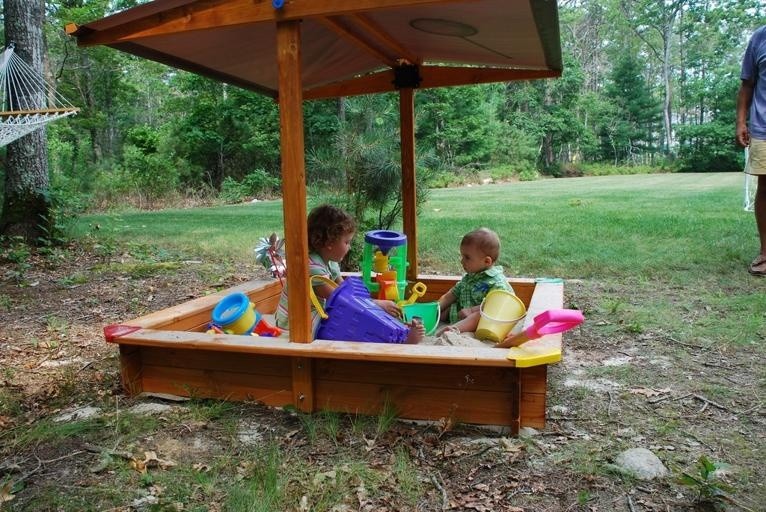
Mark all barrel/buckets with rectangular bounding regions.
[474,290,528,342]
[400,301,442,336]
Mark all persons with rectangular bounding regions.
[273,203,426,344]
[436,226,516,338]
[736,26,766,273]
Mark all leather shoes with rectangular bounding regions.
[749,254,766,277]
